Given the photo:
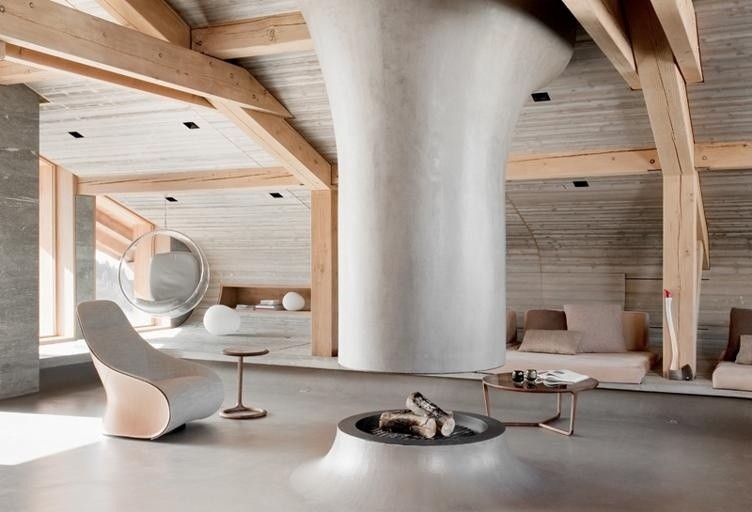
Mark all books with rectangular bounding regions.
[522,368,589,388]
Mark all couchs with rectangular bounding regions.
[711,307,751,391]
[505,309,658,384]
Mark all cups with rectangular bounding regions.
[511,371,524,387]
[527,370,536,381]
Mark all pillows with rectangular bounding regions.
[522,309,566,329]
[734,333,752,366]
[518,329,582,356]
[564,303,627,354]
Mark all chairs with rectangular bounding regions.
[78,299,225,440]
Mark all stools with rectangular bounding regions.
[221,346,270,418]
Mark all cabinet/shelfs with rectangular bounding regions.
[217,284,311,312]
